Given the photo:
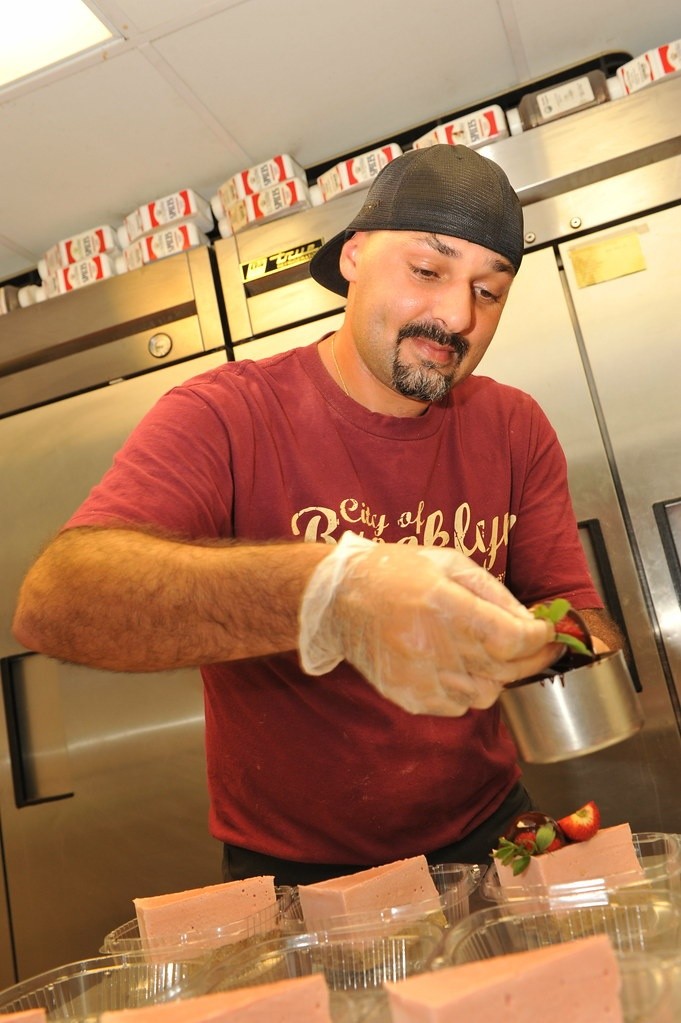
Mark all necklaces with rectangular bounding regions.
[332,335,350,398]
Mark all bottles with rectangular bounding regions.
[506,68,610,136]
[309,143,403,206]
[17,154,309,308]
[606,39,681,102]
[412,105,509,151]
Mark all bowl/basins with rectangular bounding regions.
[497,648,644,763]
[0,831,681,1023]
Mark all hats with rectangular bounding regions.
[309,144,524,298]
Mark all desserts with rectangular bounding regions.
[492,823,645,913]
[298,855,447,970]
[0,1008,47,1023]
[384,933,623,1023]
[101,973,332,1023]
[134,876,278,960]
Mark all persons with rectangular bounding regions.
[12,146,629,899]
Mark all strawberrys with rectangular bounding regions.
[556,615,585,639]
[514,800,600,857]
